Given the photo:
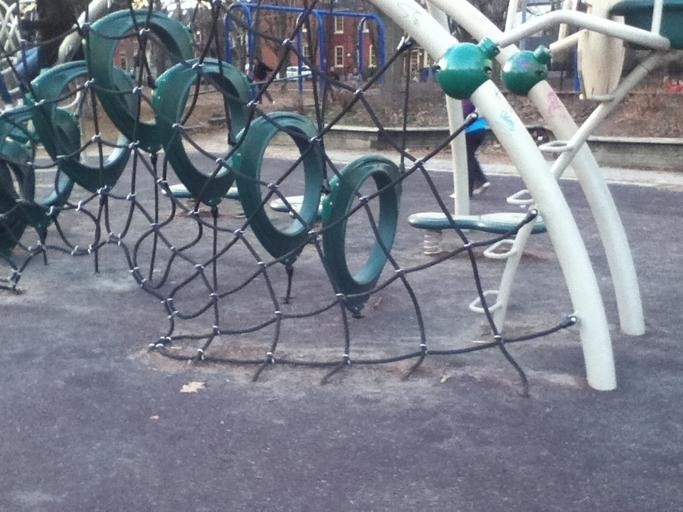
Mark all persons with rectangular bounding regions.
[449,97,491,198]
[252,56,275,104]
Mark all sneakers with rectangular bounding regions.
[472,177,490,195]
